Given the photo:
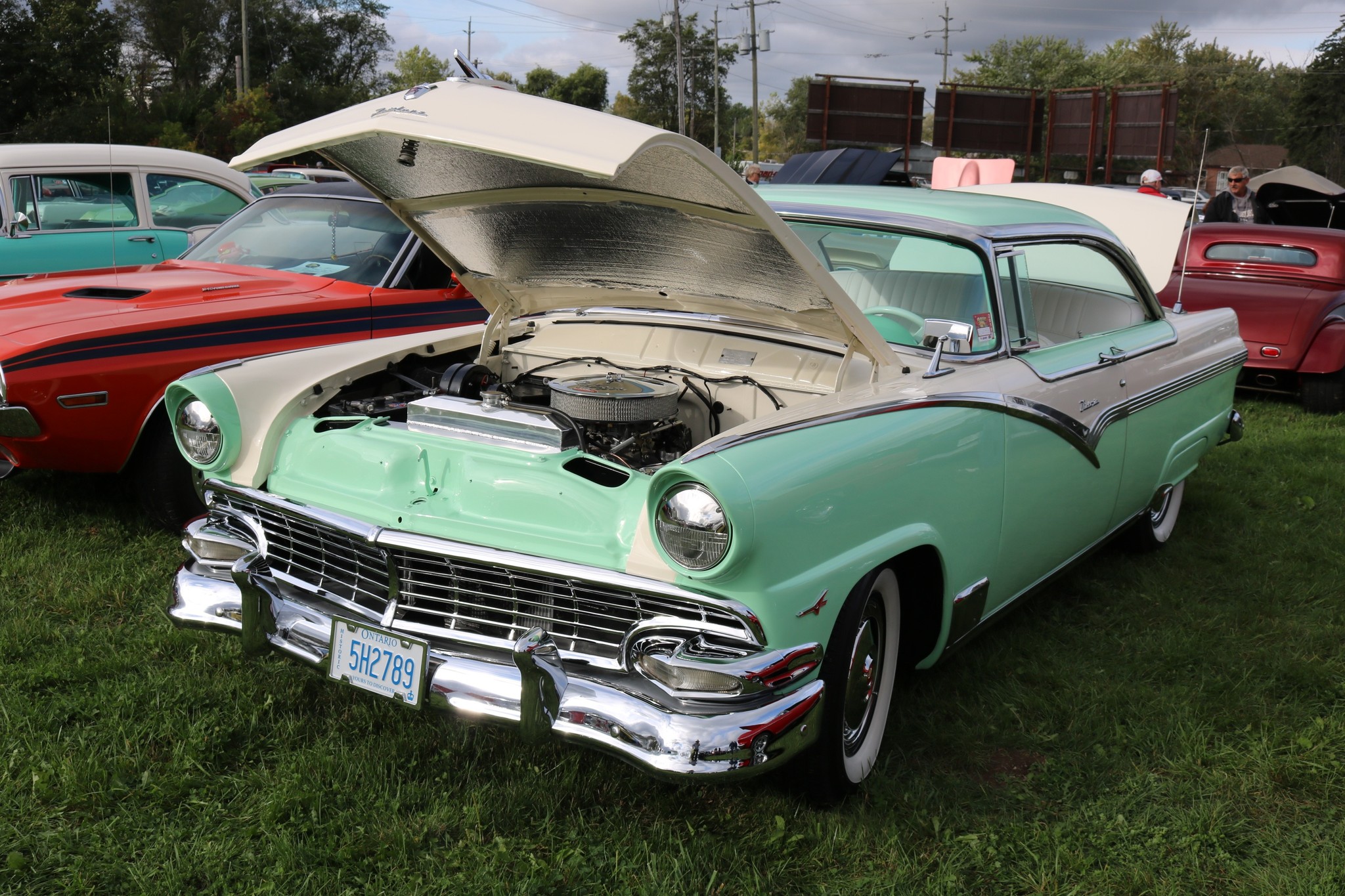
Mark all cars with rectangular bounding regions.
[0,143,527,524]
[764,146,1345,416]
[162,49,1255,796]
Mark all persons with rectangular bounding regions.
[1204,165,1268,224]
[742,163,762,185]
[1136,169,1168,199]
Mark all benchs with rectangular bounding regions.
[826,271,1143,354]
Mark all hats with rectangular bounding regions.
[1140,169,1167,184]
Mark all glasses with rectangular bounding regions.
[1227,177,1244,183]
